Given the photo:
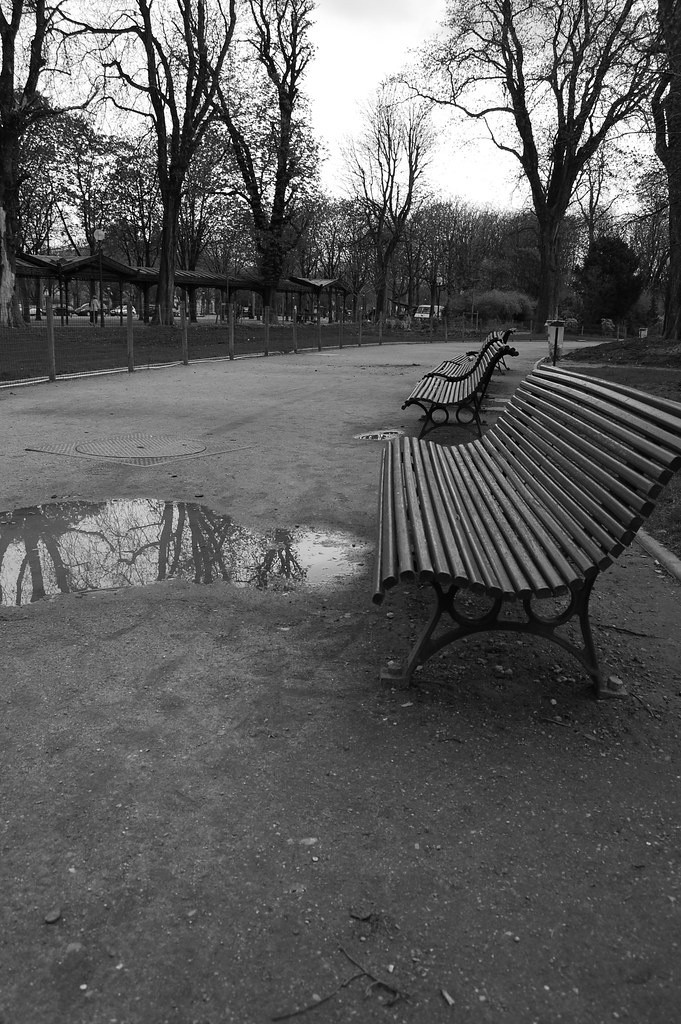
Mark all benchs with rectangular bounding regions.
[366,326,681,680]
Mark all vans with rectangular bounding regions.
[75,303,108,316]
[415,305,444,319]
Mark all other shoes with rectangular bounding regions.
[89,322,92,325]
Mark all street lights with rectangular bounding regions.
[94,229,106,327]
[434,272,446,323]
[201,289,205,316]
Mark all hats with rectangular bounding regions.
[93,295,97,298]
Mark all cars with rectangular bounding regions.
[110,304,136,316]
[40,306,75,316]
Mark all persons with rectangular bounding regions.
[89,296,100,325]
